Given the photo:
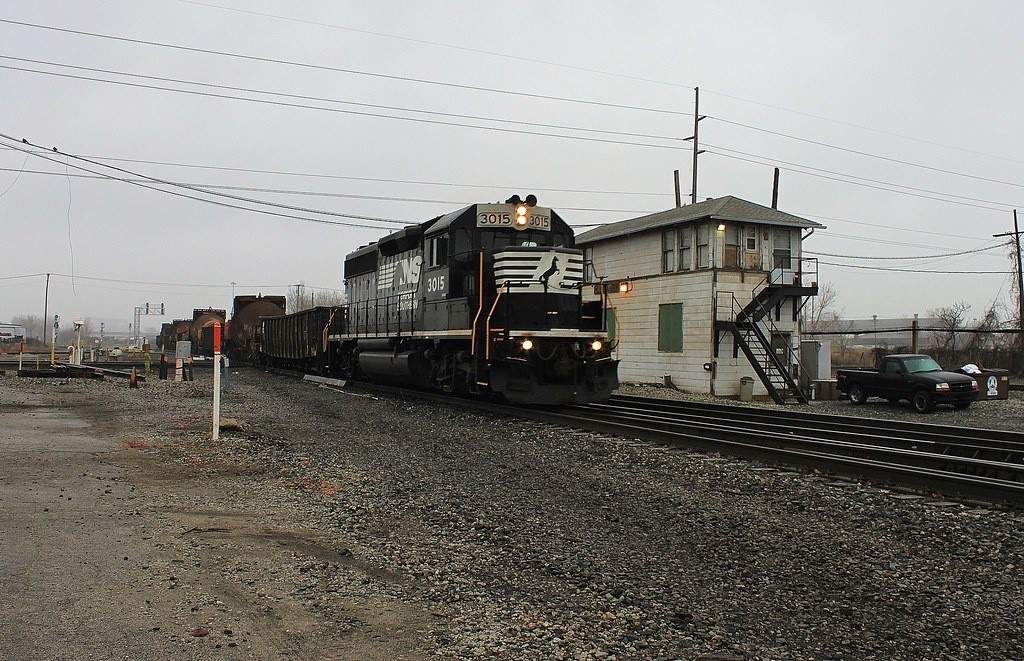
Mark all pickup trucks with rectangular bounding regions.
[834,353,980,414]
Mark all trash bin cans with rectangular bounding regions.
[740,376,755,402]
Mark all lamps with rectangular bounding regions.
[621,282,628,292]
[718,224,726,230]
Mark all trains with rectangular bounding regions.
[154,194,623,407]
